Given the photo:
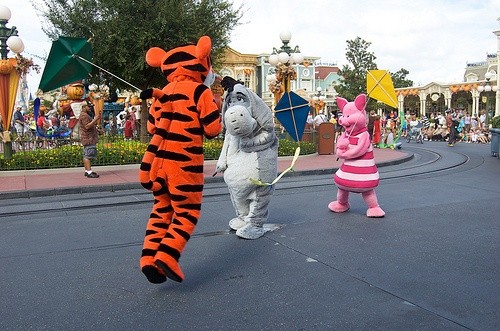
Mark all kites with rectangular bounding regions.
[274,90,311,143]
[366,69,399,109]
[39,36,92,93]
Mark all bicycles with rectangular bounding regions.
[406,128,423,143]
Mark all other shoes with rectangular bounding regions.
[87,172,99,178]
[20,146,26,149]
[448,144,453,146]
[84,172,88,176]
[429,138,491,144]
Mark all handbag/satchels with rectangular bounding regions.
[472,134,477,141]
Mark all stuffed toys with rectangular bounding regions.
[328,94,385,218]
[217,76,278,240]
[139,37,222,284]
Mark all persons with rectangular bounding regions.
[14,106,138,148]
[311,109,491,147]
[79,101,102,177]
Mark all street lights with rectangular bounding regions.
[265,65,282,131]
[312,86,326,117]
[268,27,304,93]
[0,3,27,165]
[476,71,498,141]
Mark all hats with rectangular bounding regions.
[81,100,95,106]
[16,105,22,109]
[446,110,451,113]
